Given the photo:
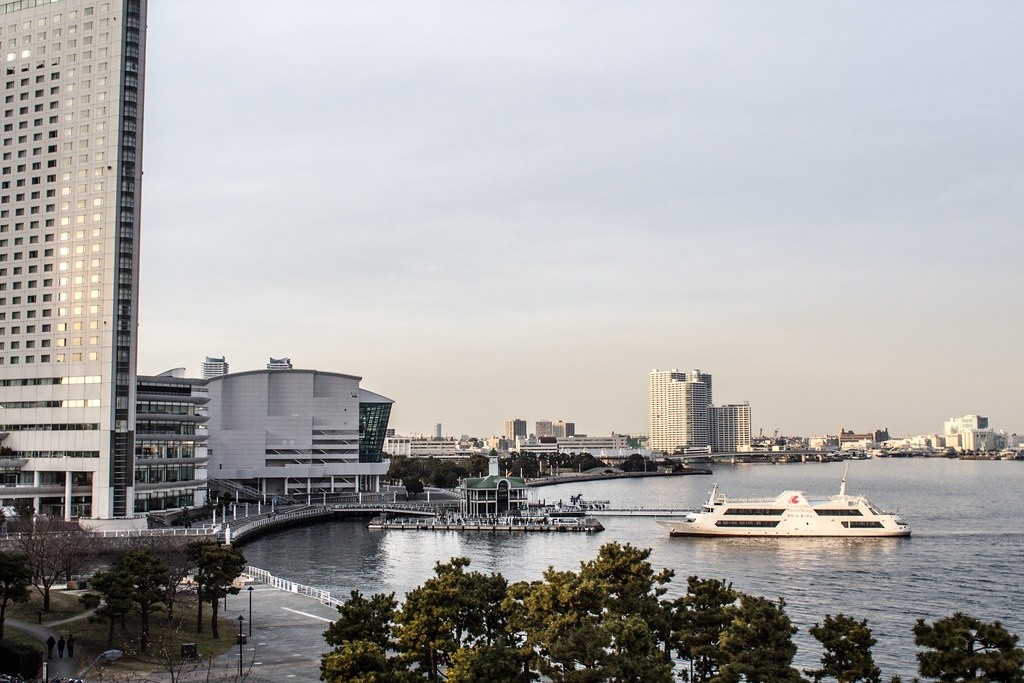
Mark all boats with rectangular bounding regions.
[655,463,913,537]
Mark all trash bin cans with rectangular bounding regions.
[182,643,199,660]
[67,580,78,590]
[79,581,87,589]
[237,633,247,644]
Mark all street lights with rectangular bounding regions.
[237,614,245,677]
[247,586,254,637]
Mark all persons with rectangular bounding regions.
[67,634,78,658]
[443,515,549,525]
[46,634,57,659]
[58,636,65,658]
